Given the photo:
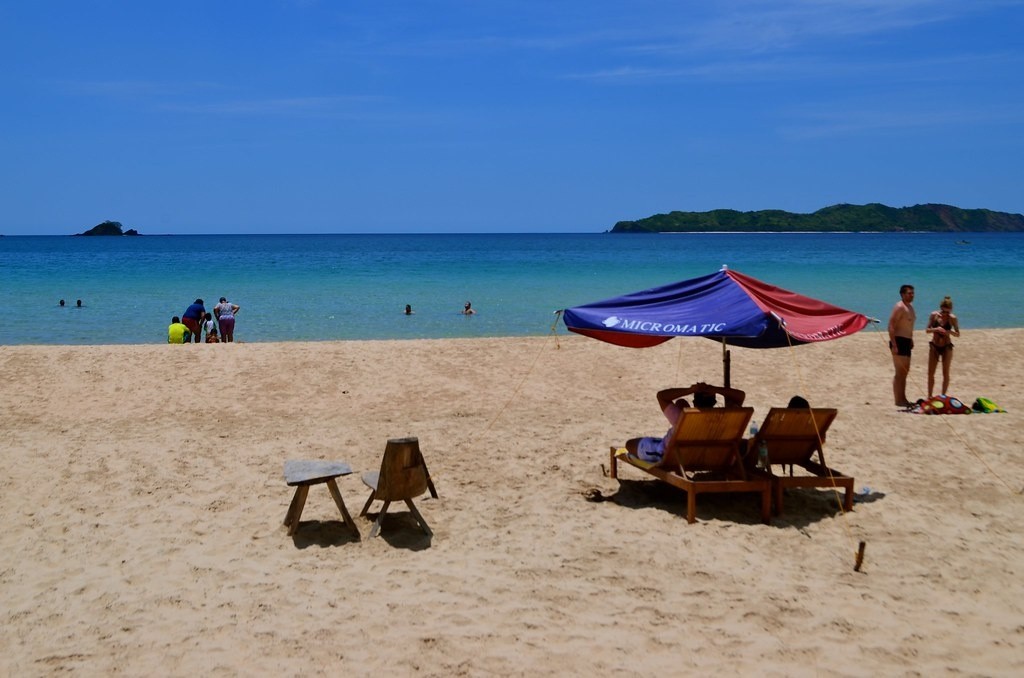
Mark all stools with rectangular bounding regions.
[281,460,359,539]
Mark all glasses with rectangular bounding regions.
[942,310,950,313]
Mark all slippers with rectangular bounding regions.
[582,489,613,501]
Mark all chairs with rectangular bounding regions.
[360,436,436,540]
[609,407,774,526]
[744,407,856,514]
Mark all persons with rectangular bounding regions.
[204,313,216,336]
[206,329,221,343]
[403,304,415,314]
[77,300,81,306]
[182,299,206,343]
[625,382,745,463]
[889,285,916,406]
[168,316,192,343]
[214,297,240,343]
[739,395,810,457]
[461,301,475,314]
[926,296,960,399]
[60,300,65,306]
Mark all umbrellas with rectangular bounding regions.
[562,264,881,407]
